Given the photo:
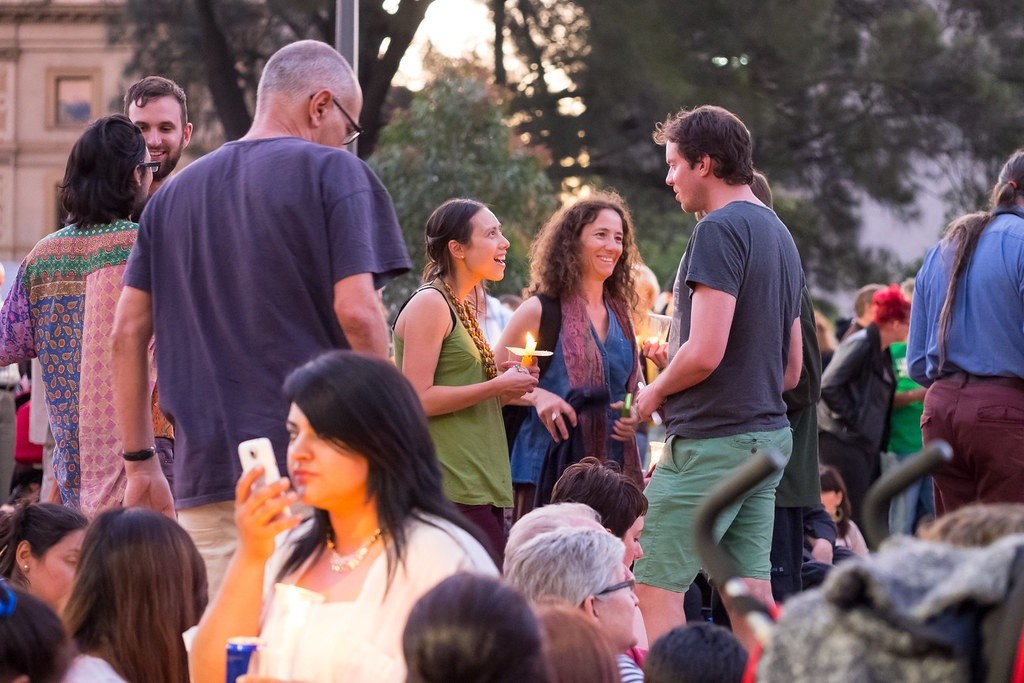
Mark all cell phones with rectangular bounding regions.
[238,437,292,521]
[621,393,632,417]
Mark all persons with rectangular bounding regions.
[0,114,175,520]
[489,196,651,524]
[695,171,821,632]
[0,263,1024,683]
[392,200,541,574]
[631,106,802,657]
[906,151,1024,512]
[122,76,193,221]
[109,37,413,611]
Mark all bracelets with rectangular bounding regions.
[121,444,157,461]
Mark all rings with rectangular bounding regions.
[550,411,560,421]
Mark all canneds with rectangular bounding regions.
[225,636,259,683]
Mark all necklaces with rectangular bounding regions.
[438,274,499,380]
[326,527,383,578]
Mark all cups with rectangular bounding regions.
[645,312,672,345]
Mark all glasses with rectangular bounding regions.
[309,92,364,145]
[600,579,635,595]
[138,161,161,173]
